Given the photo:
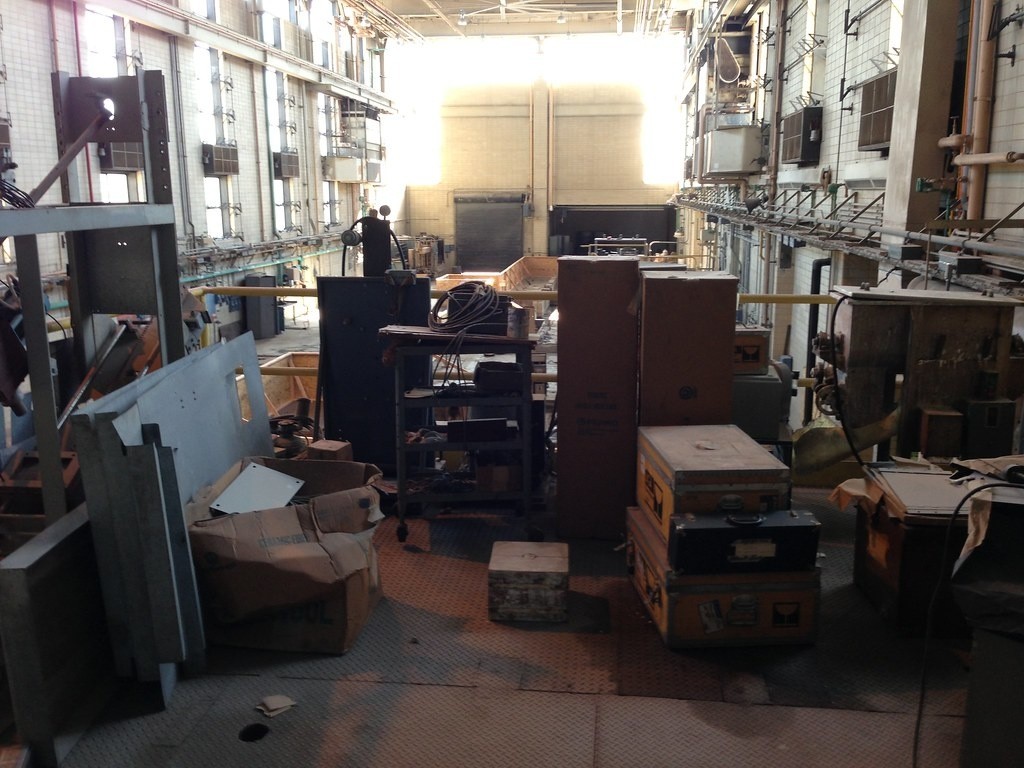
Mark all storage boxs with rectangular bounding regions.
[488,541,570,623]
[184,454,381,656]
[854,501,975,641]
[915,406,963,457]
[635,425,791,546]
[736,325,770,375]
[308,440,354,462]
[557,256,639,542]
[962,396,1015,459]
[641,270,740,425]
[626,508,819,649]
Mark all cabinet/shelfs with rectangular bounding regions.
[393,342,545,542]
